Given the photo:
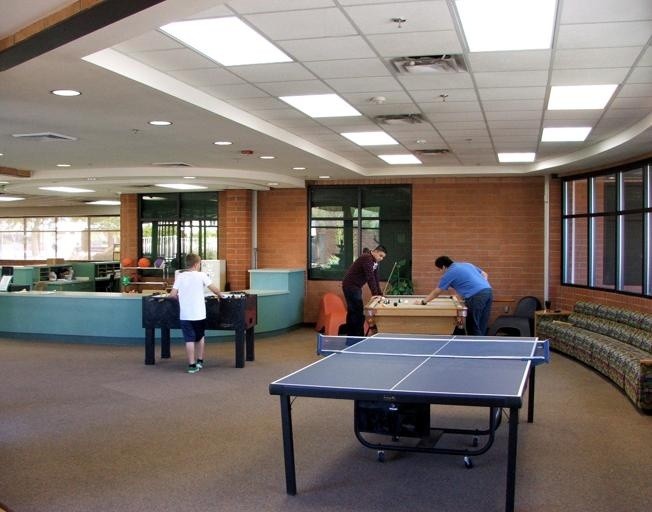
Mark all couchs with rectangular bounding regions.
[535,299,651,415]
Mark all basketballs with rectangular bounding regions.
[138,258,151,268]
[121,258,134,268]
[154,257,166,268]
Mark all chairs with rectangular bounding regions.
[487,296,542,336]
[314,293,372,336]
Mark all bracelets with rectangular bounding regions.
[422,299,427,305]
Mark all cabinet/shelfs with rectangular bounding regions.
[0,261,120,291]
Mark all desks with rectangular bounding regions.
[268,331,549,512]
[363,295,463,334]
[141,290,258,369]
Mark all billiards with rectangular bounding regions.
[394,302,398,306]
[381,296,392,307]
[398,298,403,304]
[404,300,409,304]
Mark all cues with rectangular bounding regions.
[365,262,398,336]
[418,300,466,303]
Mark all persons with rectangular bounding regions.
[167,253,226,374]
[341,245,389,346]
[414,256,494,336]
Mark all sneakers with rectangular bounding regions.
[188,362,203,373]
